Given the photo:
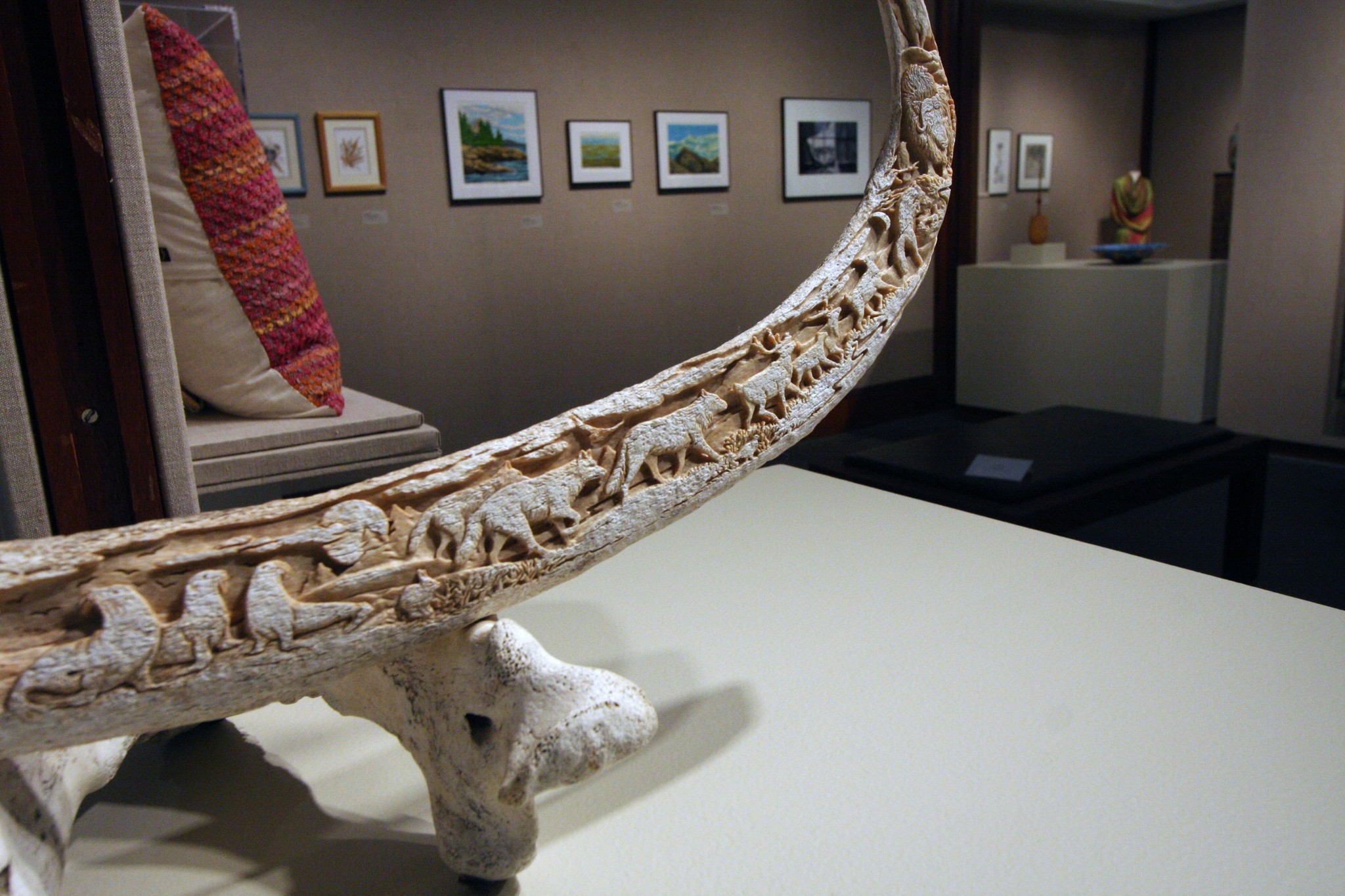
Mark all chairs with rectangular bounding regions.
[0,0,443,543]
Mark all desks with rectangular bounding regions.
[956,256,1228,426]
[60,464,1345,896]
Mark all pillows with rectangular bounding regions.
[123,0,346,422]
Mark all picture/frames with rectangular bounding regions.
[565,119,634,187]
[248,112,308,198]
[315,111,388,197]
[654,109,731,191]
[780,97,874,203]
[1015,132,1053,192]
[986,126,1013,198]
[437,87,546,205]
